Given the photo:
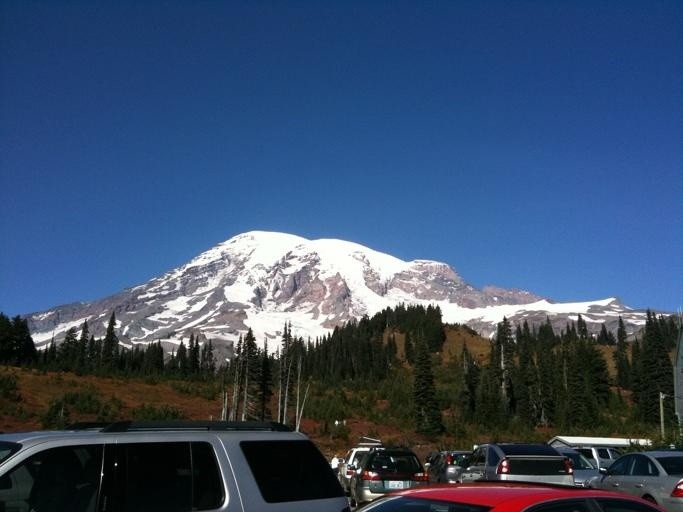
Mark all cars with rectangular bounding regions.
[583,450,682,511]
[350,483,671,512]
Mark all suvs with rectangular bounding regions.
[421,442,624,490]
[335,443,385,497]
[346,445,427,508]
[0,420,351,512]
